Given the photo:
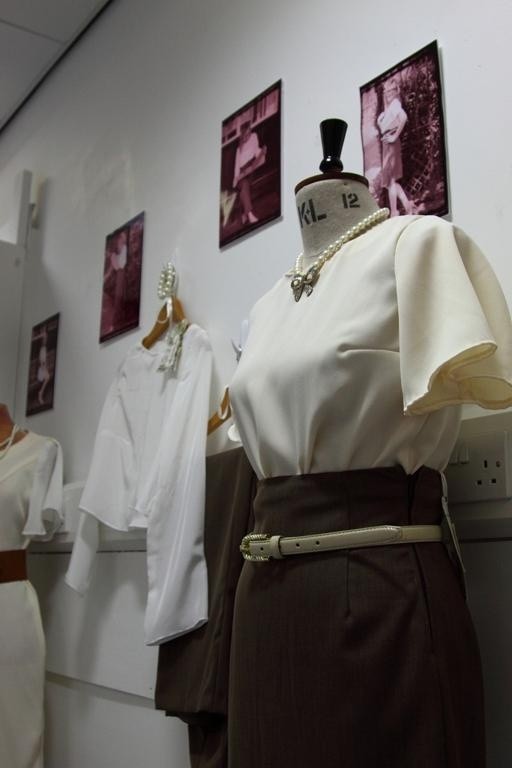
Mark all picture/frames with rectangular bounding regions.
[358,38,450,217]
[218,77,282,249]
[23,311,61,415]
[98,210,144,344]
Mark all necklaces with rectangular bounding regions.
[290,207,390,301]
[0,422,22,459]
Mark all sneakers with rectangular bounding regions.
[242,211,259,226]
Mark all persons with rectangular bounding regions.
[31,321,50,405]
[375,77,415,218]
[225,114,511,768]
[102,231,138,310]
[231,120,267,223]
[0,401,65,768]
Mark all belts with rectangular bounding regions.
[238,521,446,565]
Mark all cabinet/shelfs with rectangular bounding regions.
[120,292,211,369]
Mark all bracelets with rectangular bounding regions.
[157,261,175,299]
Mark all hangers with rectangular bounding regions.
[208,385,233,434]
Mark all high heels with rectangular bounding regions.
[390,200,417,217]
